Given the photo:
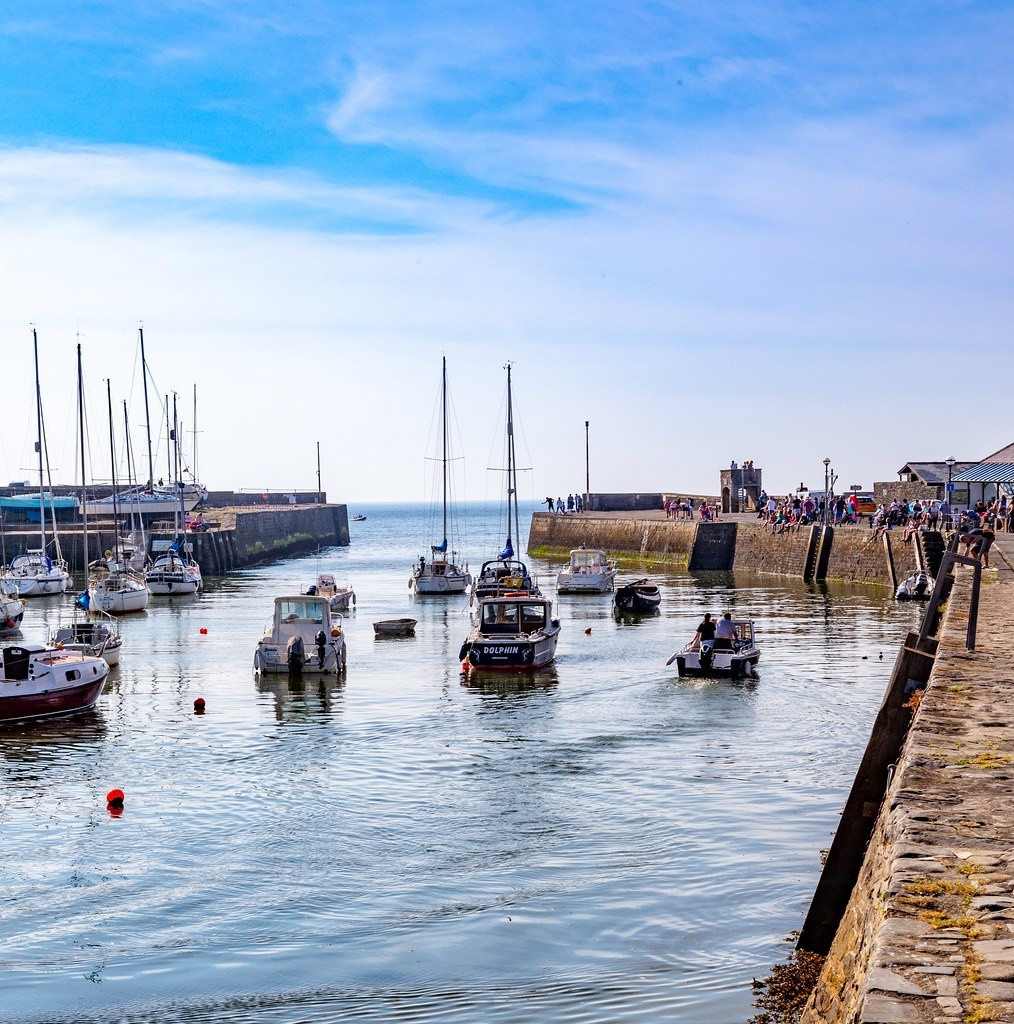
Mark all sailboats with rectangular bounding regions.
[467,360,542,602]
[0,322,204,669]
[405,355,475,594]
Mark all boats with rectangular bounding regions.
[349,516,367,521]
[459,574,563,671]
[294,573,357,612]
[894,569,937,600]
[254,594,348,674]
[611,577,661,615]
[0,642,110,724]
[666,617,762,680]
[556,541,618,593]
[373,619,418,635]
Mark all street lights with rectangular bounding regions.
[822,457,831,526]
[945,456,956,511]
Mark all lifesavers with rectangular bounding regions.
[505,591,529,598]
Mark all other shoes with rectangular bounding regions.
[981,565,989,569]
[1001,527,1004,530]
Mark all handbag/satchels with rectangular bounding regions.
[999,507,1005,516]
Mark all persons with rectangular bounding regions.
[976,495,1014,532]
[541,497,555,514]
[828,495,854,527]
[761,490,767,496]
[556,497,565,514]
[714,613,737,654]
[730,461,737,469]
[159,478,163,486]
[870,498,981,542]
[195,512,204,524]
[765,493,825,535]
[568,493,583,514]
[664,497,714,520]
[691,613,716,649]
[960,528,995,570]
[743,460,753,469]
[185,513,191,529]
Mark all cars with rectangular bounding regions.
[907,499,943,514]
[854,496,877,516]
